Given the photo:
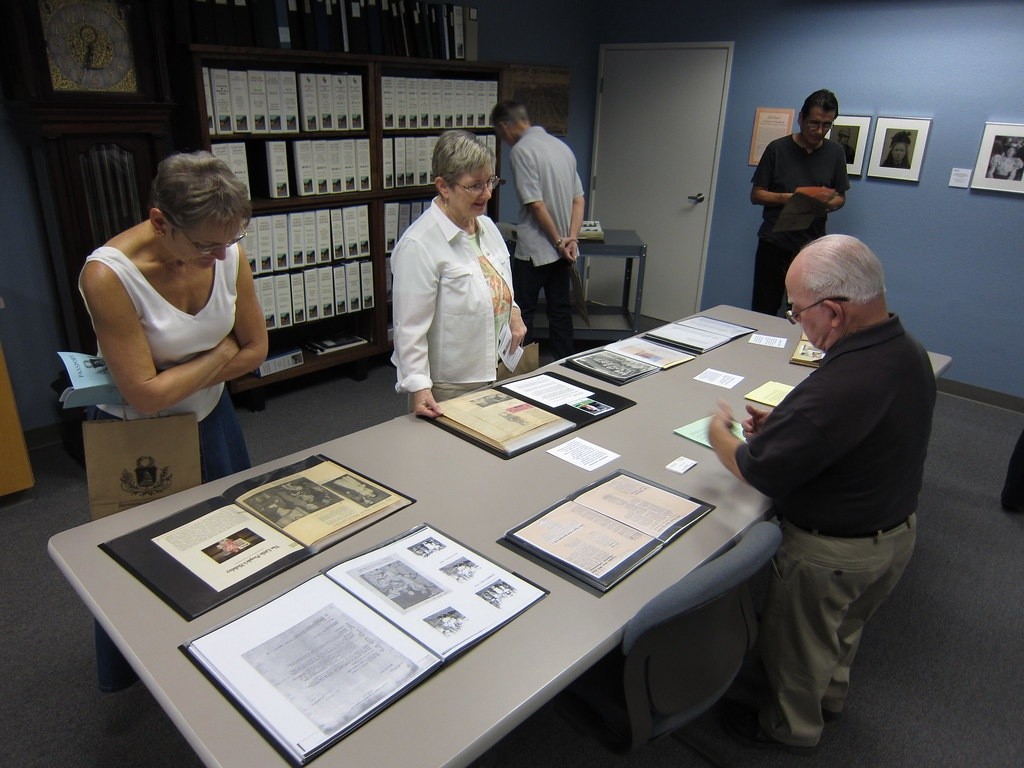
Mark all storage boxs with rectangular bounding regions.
[199,64,499,378]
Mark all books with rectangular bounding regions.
[56,351,128,409]
[495,467,717,598]
[789,332,826,368]
[558,337,697,387]
[418,371,637,460]
[97,452,417,623]
[641,315,758,354]
[177,523,551,768]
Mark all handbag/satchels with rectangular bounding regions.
[82,396,202,522]
[497,336,539,383]
[567,262,590,327]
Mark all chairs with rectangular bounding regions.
[557,521,784,768]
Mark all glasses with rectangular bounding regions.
[442,174,501,194]
[782,295,847,328]
[159,208,247,255]
[802,117,834,131]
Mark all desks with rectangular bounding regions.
[507,229,649,342]
[49,304,952,768]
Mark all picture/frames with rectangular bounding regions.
[967,121,1024,193]
[821,114,873,175]
[867,116,932,181]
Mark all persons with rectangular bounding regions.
[581,403,600,412]
[881,130,912,169]
[79,150,268,486]
[837,127,855,164]
[491,98,585,360]
[749,89,850,319]
[987,141,1023,180]
[708,232,938,754]
[390,131,527,420]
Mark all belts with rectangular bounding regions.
[785,515,910,538]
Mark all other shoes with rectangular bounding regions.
[721,702,820,757]
[741,669,843,722]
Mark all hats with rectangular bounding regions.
[838,128,850,137]
[1003,140,1023,149]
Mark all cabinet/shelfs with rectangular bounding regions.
[184,40,504,394]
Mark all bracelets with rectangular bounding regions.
[554,237,562,248]
